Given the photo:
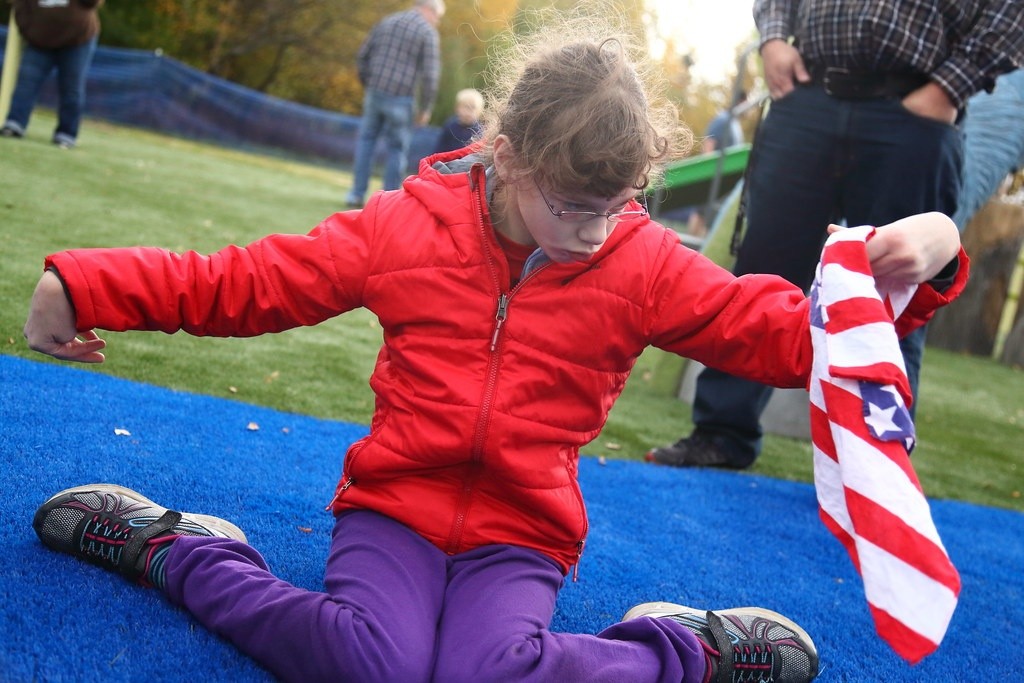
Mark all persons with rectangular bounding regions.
[21,10,969,683]
[346,0,446,207]
[434,87,486,157]
[897,65,1023,421]
[689,207,716,237]
[642,1,1024,471]
[0,0,105,148]
[702,91,747,153]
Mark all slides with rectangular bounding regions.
[635,142,753,217]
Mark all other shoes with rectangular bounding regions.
[0,126,20,138]
[54,137,72,146]
[645,431,728,467]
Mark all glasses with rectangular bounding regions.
[532,176,648,224]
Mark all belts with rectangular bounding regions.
[809,65,922,96]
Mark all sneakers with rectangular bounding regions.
[33,484,248,589]
[621,601,819,683]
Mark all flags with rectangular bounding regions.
[807,224,961,666]
